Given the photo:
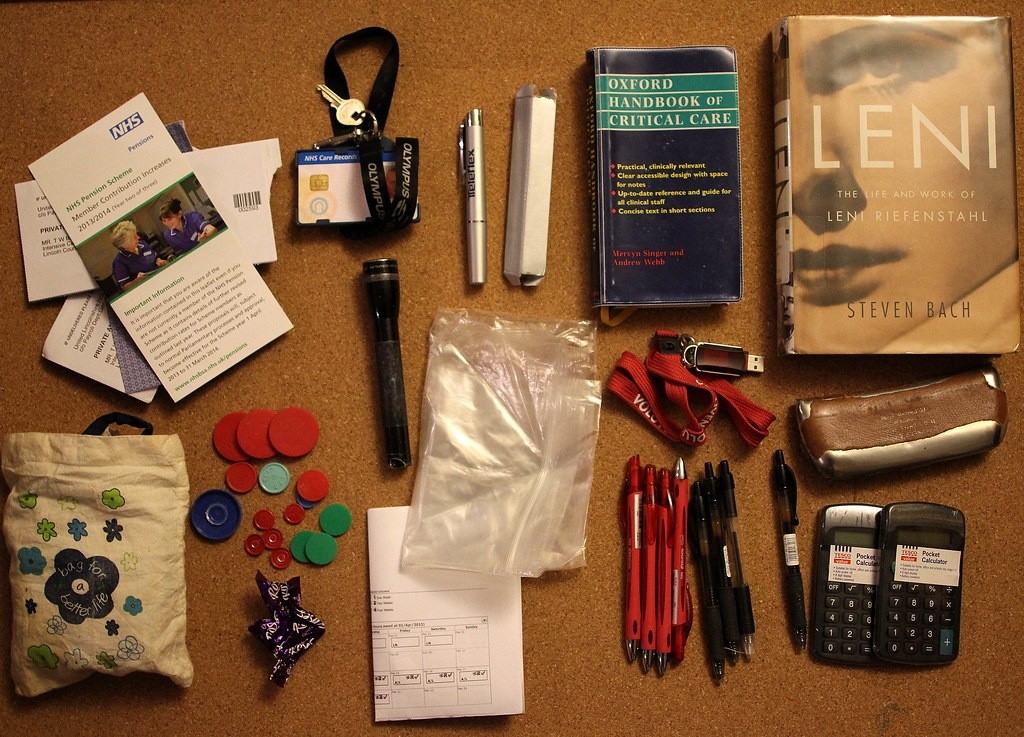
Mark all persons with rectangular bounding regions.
[159,197,218,256]
[111,220,169,292]
[792,19,1019,350]
[387,164,399,200]
[775,25,789,63]
[780,282,795,353]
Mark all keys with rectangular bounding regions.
[313,84,381,150]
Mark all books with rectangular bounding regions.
[773,13,1021,354]
[583,46,743,306]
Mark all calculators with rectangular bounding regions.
[809,499,882,667]
[875,498,968,668]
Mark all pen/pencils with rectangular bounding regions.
[457,107,489,287]
[618,452,754,684]
[773,448,807,653]
